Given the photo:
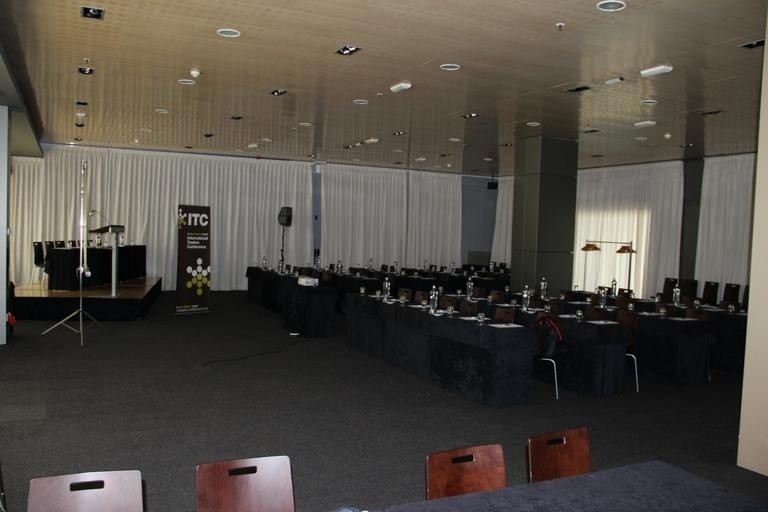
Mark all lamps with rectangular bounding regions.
[88,209,123,245]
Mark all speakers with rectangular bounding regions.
[278,207,293,227]
[487,181,498,190]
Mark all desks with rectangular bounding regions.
[43,245,146,291]
[244,264,744,410]
[370,459,768,512]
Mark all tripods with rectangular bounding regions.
[39,161,112,348]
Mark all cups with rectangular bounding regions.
[360,264,735,323]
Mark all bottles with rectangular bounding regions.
[261,256,343,275]
[424,255,428,272]
[394,258,401,277]
[369,258,374,273]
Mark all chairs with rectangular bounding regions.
[425,444,507,501]
[286,264,749,400]
[27,469,144,512]
[194,454,295,512]
[30,240,93,290]
[528,426,591,484]
